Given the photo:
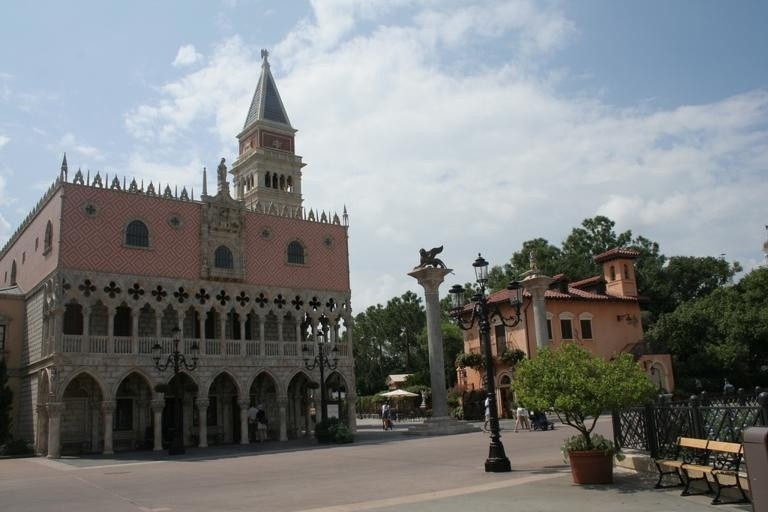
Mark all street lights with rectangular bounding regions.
[296,307,342,428]
[443,250,526,475]
[148,323,203,456]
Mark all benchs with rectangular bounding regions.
[652,435,752,505]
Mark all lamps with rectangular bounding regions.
[617,313,631,322]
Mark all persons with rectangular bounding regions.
[254,403,268,443]
[483,397,491,432]
[512,407,533,432]
[247,403,260,442]
[381,399,391,430]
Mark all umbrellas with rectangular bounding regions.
[378,388,421,423]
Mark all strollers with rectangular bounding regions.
[526,406,555,431]
[381,415,393,431]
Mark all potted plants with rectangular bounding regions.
[508,339,656,487]
